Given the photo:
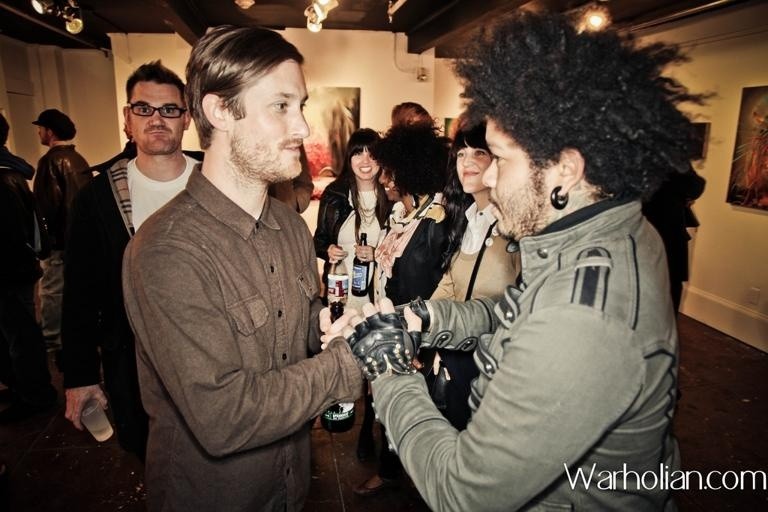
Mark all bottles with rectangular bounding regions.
[327,247,348,306]
[351,233,370,297]
[319,301,356,432]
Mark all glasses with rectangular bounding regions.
[128,102,186,118]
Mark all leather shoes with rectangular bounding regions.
[353,476,384,497]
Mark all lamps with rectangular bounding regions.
[303,1,340,32]
[417,68,427,81]
[235,0,255,10]
[30,0,83,35]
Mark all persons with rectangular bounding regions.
[0,15,681,512]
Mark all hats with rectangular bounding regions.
[32,109,75,141]
